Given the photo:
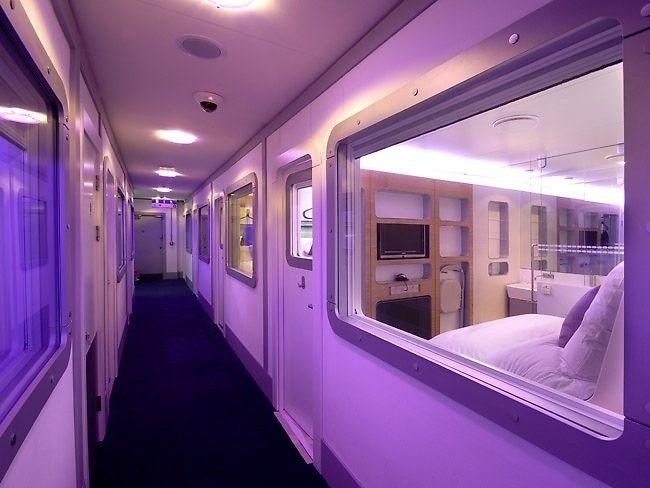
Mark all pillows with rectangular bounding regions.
[557,260,625,384]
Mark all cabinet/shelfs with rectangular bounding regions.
[472,185,521,322]
[521,192,556,273]
[369,171,473,330]
[556,196,623,275]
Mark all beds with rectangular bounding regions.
[428,311,598,400]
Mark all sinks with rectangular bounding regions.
[506,283,533,302]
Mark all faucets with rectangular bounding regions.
[534,274,543,280]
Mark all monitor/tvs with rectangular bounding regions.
[380,223,426,258]
